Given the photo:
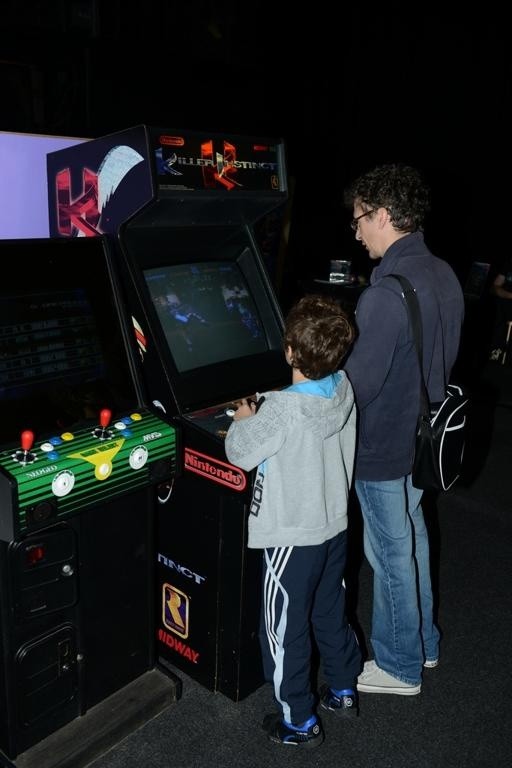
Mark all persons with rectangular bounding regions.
[344,161,467,697]
[224,293,363,748]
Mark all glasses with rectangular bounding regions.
[351,208,394,231]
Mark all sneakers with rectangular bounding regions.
[354,659,421,696]
[317,688,359,719]
[262,711,323,747]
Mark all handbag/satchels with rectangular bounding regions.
[411,378,467,491]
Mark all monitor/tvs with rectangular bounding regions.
[0,249,133,452]
[130,242,293,414]
